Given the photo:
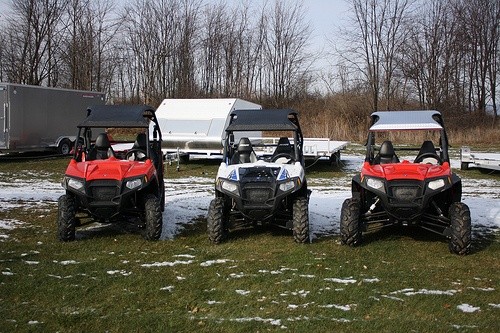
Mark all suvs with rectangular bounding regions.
[334,108,476,258]
[206,110,312,243]
[55,105,166,240]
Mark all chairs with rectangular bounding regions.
[373,140,400,166]
[413,141,441,164]
[273,137,295,163]
[88,133,115,160]
[233,137,258,163]
[128,133,157,165]
